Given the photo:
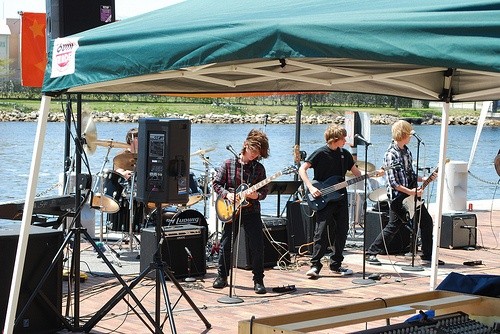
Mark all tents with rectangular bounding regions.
[3,0,500,334]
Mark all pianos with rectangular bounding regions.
[0,192,84,229]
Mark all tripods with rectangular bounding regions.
[52,92,364,334]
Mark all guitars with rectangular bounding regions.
[215,165,298,224]
[401,158,450,221]
[305,165,391,211]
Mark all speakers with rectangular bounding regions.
[287,201,334,255]
[440,213,477,248]
[140,225,207,280]
[136,118,191,204]
[45,0,116,39]
[233,218,291,269]
[0,219,62,334]
[366,210,411,255]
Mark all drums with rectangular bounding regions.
[148,202,169,209]
[88,168,127,214]
[106,195,148,235]
[363,170,389,202]
[196,177,211,201]
[144,205,208,246]
[177,171,203,208]
[345,189,365,224]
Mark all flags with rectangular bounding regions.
[18,11,46,87]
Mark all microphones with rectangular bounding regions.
[459,225,475,229]
[355,134,370,145]
[185,246,193,257]
[225,144,240,160]
[410,130,425,146]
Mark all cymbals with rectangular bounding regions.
[91,139,132,148]
[345,170,357,178]
[113,152,138,172]
[191,147,215,157]
[355,161,375,172]
[81,108,97,156]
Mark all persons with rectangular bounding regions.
[112,127,138,179]
[298,123,385,278]
[364,120,445,266]
[213,129,270,294]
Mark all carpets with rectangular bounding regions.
[344,228,365,250]
[64,247,140,278]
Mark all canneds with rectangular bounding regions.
[469,204,472,210]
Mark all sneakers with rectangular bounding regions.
[365,253,380,264]
[306,265,320,276]
[254,280,266,293]
[420,255,445,264]
[213,276,228,287]
[329,266,353,274]
